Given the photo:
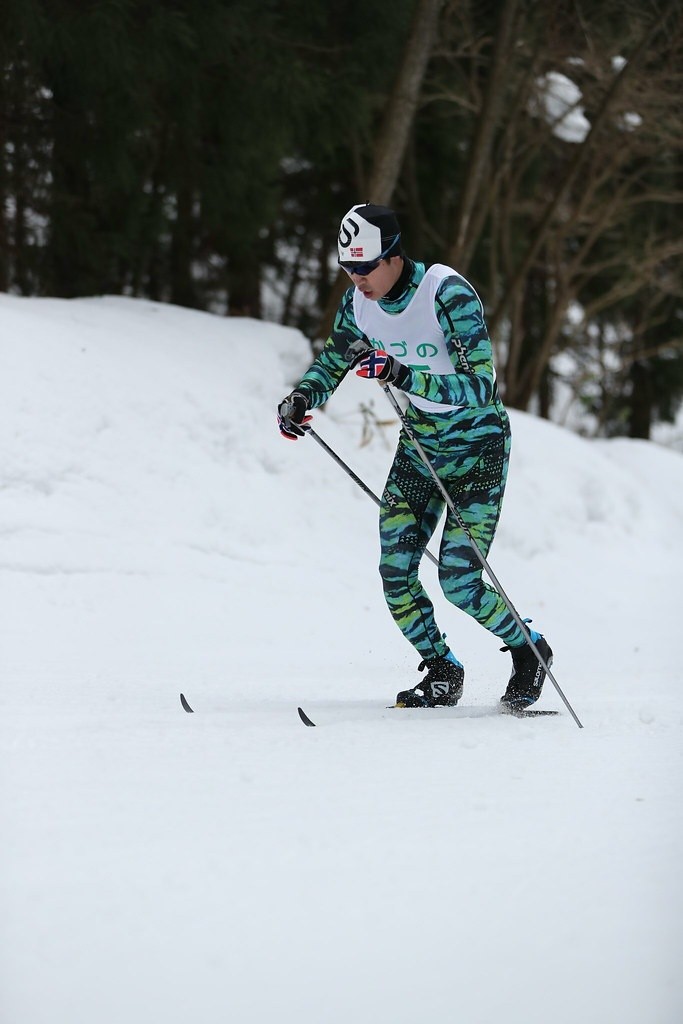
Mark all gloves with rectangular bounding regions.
[356,349,410,389]
[276,388,313,440]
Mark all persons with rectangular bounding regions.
[278,203,553,710]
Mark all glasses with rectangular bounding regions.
[338,235,399,276]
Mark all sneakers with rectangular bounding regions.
[500,619,553,710]
[396,633,465,707]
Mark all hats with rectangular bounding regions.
[338,203,402,261]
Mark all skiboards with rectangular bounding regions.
[176,690,562,730]
